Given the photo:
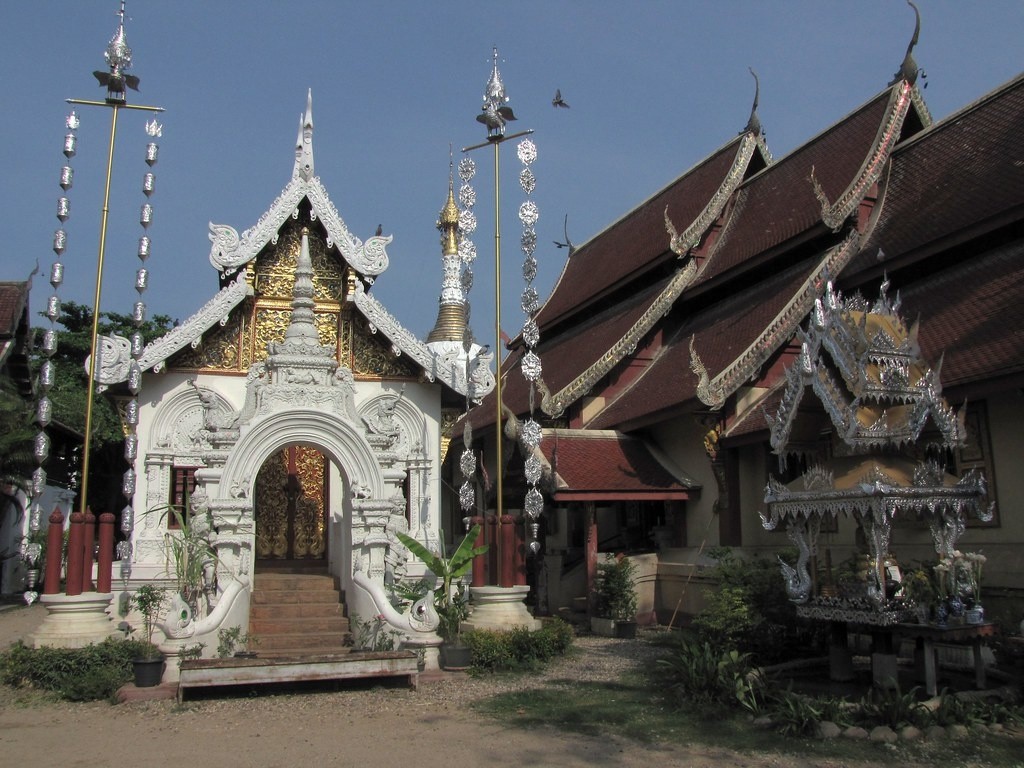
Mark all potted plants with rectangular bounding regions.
[590,552,638,639]
[126,584,166,688]
[215,624,261,659]
[436,584,472,666]
[343,612,375,653]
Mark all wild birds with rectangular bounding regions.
[375,224,383,236]
[173,318,179,328]
[552,88,569,109]
